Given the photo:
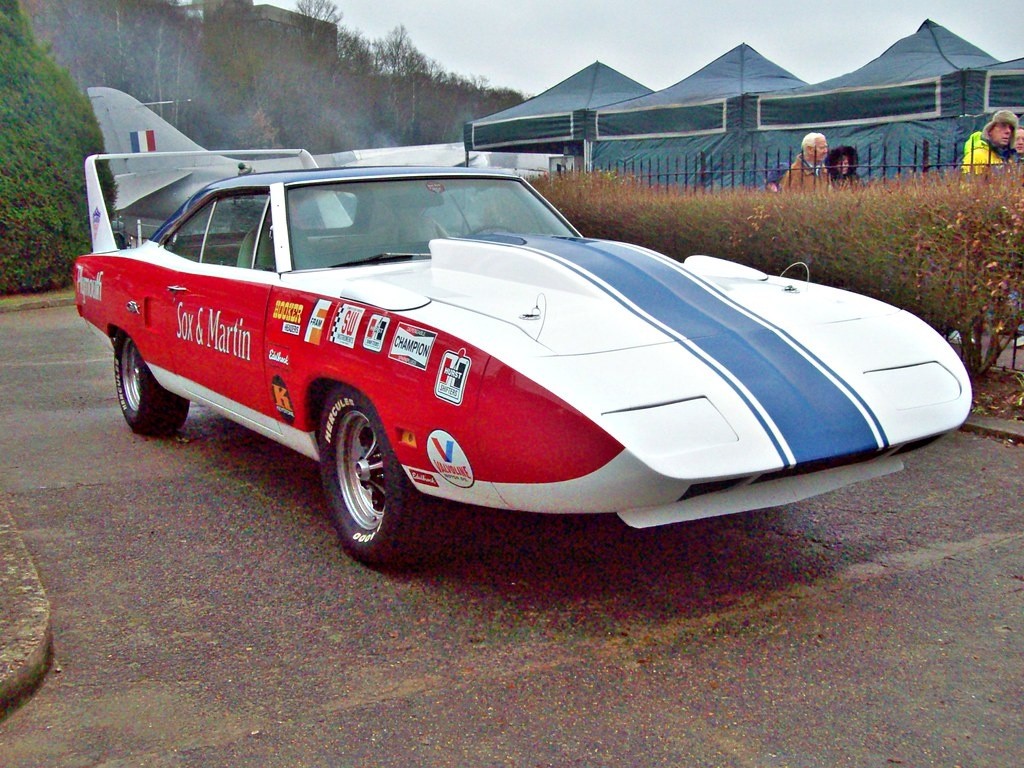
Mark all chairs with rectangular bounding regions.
[235,221,331,266]
[371,198,450,244]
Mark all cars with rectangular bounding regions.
[71,148,972,571]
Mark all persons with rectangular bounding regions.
[781,133,833,194]
[758,163,791,194]
[825,145,864,189]
[959,110,1021,179]
[1013,126,1024,178]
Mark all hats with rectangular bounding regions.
[981,109,1019,149]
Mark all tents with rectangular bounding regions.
[463,19,1024,193]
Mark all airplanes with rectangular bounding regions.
[87,87,581,245]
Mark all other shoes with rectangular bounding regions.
[1011,335,1024,347]
[942,330,975,345]
